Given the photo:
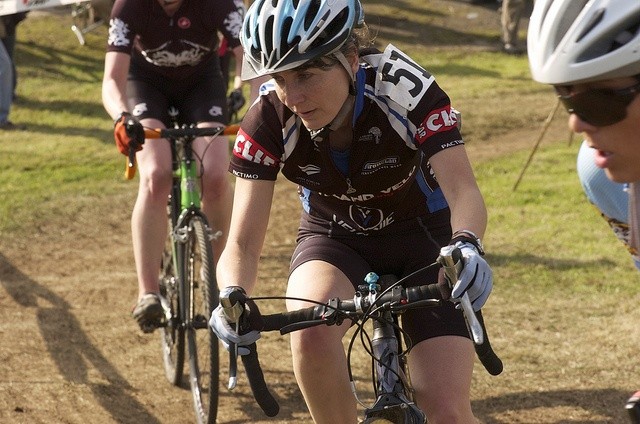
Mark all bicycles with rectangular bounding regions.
[218,246,504,424]
[123,117,242,424]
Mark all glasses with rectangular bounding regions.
[558,86,640,127]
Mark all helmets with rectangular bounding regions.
[525,1,640,86]
[238,0,364,81]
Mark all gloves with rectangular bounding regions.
[437,230,495,314]
[112,111,146,156]
[208,285,261,356]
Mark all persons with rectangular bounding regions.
[102,0,273,328]
[208,0,493,424]
[527,0,640,271]
[0,0,31,129]
[501,0,533,54]
[218,0,246,109]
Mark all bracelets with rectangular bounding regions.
[452,230,480,246]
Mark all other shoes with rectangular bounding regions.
[131,293,166,330]
